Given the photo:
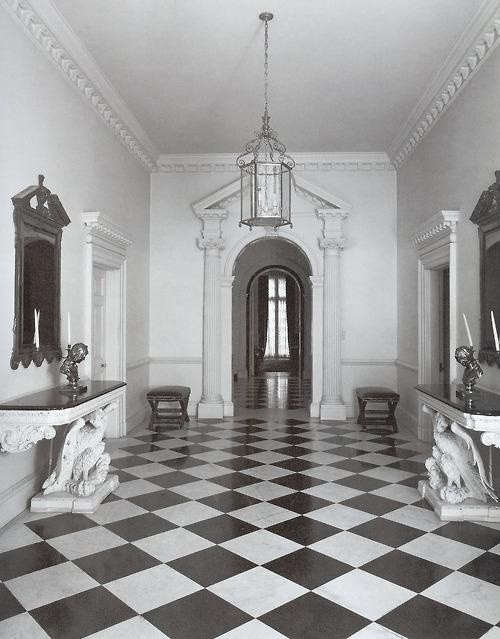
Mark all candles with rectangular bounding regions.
[32,312,40,344]
[490,311,500,351]
[34,308,40,348]
[462,312,473,347]
[68,311,72,348]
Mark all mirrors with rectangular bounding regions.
[469,170,500,371]
[10,173,75,371]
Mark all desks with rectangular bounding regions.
[0,381,127,515]
[413,383,500,523]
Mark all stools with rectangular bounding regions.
[356,385,400,432]
[146,385,193,429]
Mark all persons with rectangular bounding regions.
[59,343,88,387]
[454,346,483,391]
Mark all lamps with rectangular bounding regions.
[237,13,297,231]
